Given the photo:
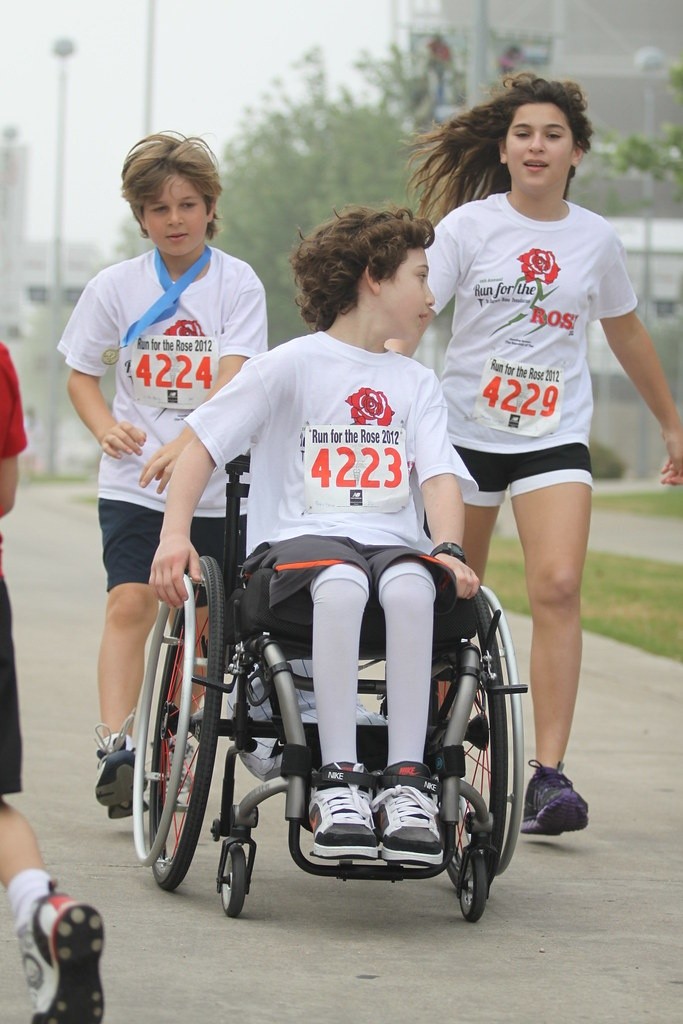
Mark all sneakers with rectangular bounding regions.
[370,762,443,867]
[308,762,379,860]
[520,760,589,836]
[18,880,105,1024]
[93,714,148,819]
[164,743,196,812]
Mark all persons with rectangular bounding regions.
[55,131,271,814]
[1,333,110,1024]
[406,70,682,837]
[150,205,482,866]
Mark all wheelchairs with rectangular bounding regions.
[130,452,527,924]
